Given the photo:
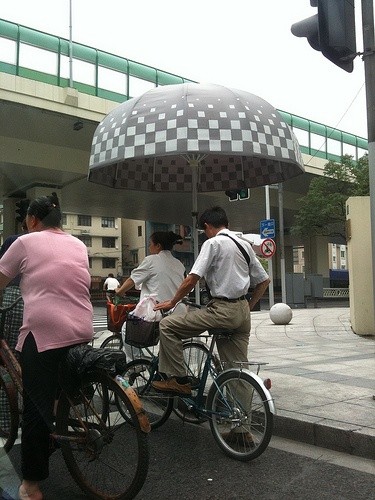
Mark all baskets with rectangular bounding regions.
[125,313,162,349]
[105,303,136,333]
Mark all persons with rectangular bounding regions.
[150,205,272,450]
[0,192,95,500]
[117,231,195,376]
[102,272,121,304]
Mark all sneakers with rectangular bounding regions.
[220,431,255,447]
[151,377,192,397]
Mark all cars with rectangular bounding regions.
[189,285,210,306]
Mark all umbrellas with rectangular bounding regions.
[86,81,308,379]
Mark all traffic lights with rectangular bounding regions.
[291,0,356,74]
[225,188,251,202]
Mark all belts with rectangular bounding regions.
[216,296,246,303]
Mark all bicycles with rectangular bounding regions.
[104,290,115,305]
[100,288,224,424]
[115,298,277,462]
[0,295,153,500]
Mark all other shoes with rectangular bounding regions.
[0,485,22,500]
